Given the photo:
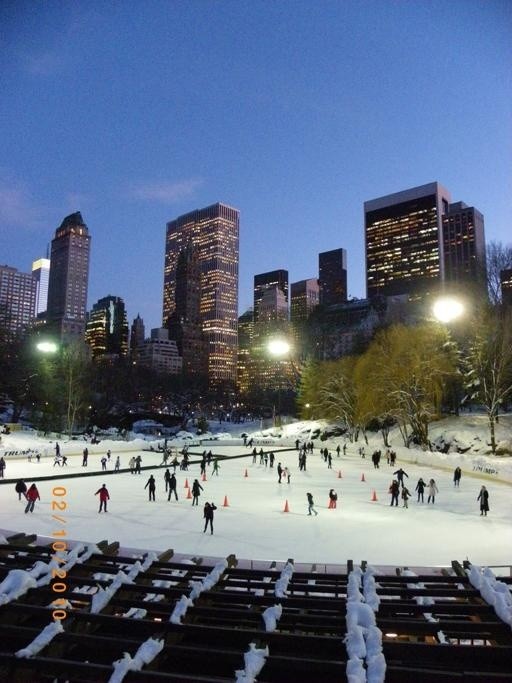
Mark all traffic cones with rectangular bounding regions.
[371,490,379,501]
[183,477,190,488]
[223,495,229,506]
[360,472,366,481]
[186,487,193,499]
[200,470,207,481]
[282,499,291,512]
[337,470,343,478]
[244,468,248,477]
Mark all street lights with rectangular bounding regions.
[265,330,295,425]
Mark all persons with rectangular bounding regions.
[453,467,461,485]
[477,486,489,515]
[203,502,217,535]
[1,433,439,514]
